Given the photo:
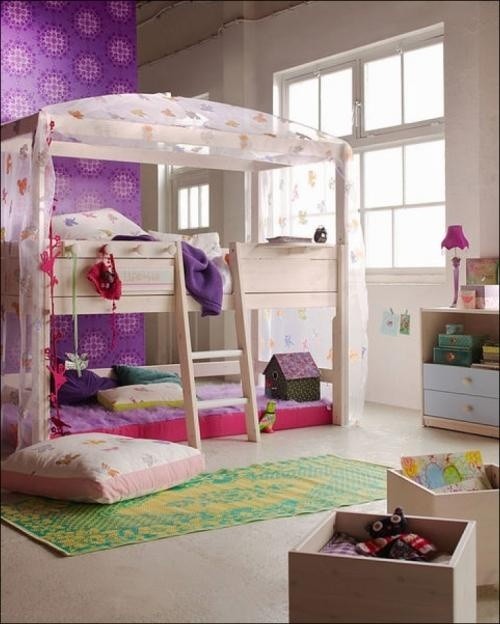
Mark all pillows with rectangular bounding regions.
[112,363,184,388]
[51,206,150,243]
[2,433,205,504]
[97,381,187,414]
[147,229,221,257]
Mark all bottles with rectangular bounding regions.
[314,228,327,243]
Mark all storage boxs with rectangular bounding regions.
[388,463,500,586]
[287,510,476,623]
[461,285,500,309]
[433,333,490,367]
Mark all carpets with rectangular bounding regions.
[2,453,397,557]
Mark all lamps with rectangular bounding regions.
[441,225,470,308]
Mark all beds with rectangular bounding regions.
[0,92,347,456]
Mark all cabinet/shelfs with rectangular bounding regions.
[420,307,500,439]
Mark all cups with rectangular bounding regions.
[459,290,475,308]
